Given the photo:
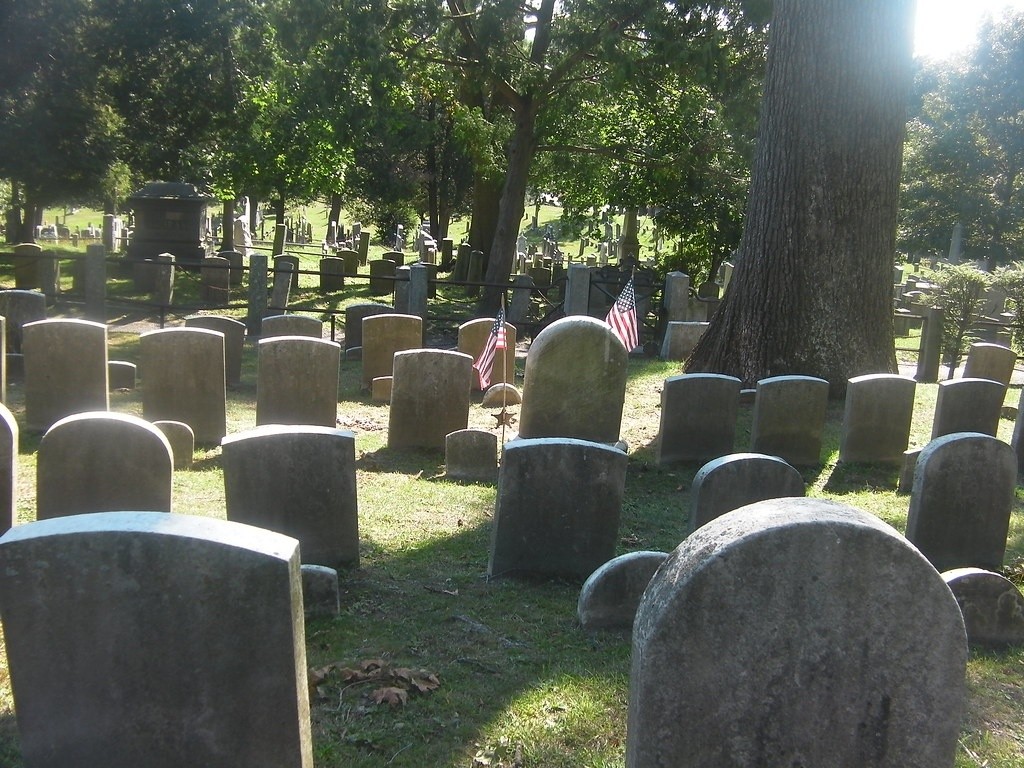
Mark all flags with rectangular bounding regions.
[604,275,638,354]
[472,306,508,391]
